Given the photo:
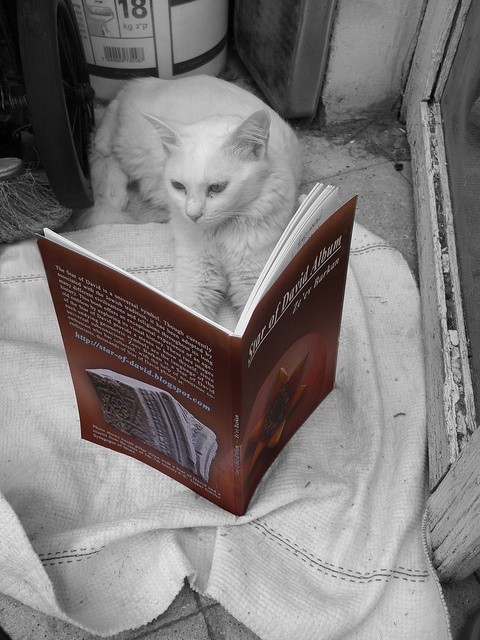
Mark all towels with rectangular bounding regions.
[0,192,456,640]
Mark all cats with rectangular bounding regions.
[73,73,305,326]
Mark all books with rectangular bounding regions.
[34,183,372,515]
